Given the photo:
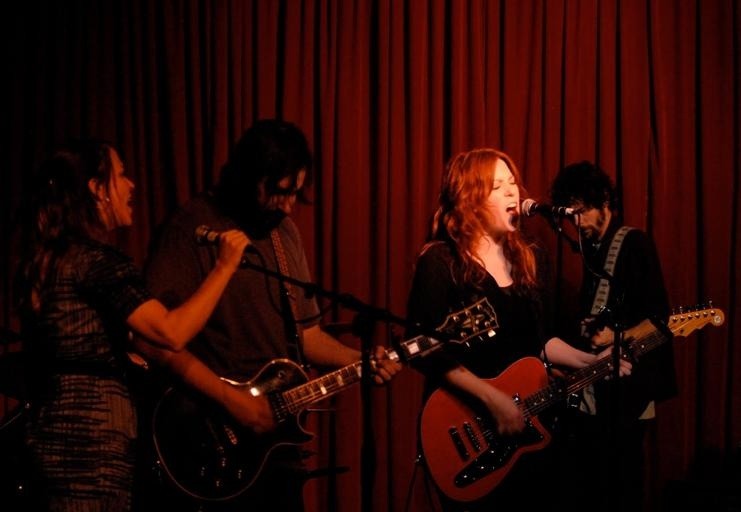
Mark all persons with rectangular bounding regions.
[138,118,403,512]
[551,160,676,512]
[12,130,254,512]
[404,149,633,512]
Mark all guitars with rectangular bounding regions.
[152,297,499,502]
[421,300,725,501]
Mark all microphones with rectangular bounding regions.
[521,198,577,218]
[194,225,253,254]
